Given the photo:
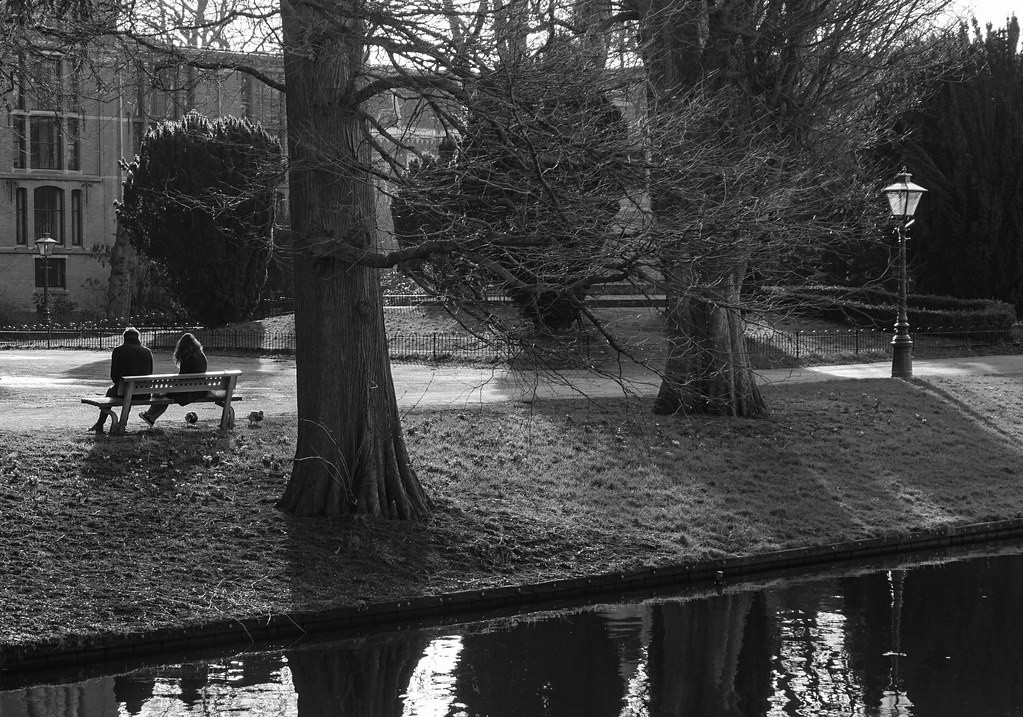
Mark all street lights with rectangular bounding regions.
[35,233,58,326]
[882,166,928,378]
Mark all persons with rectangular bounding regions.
[87,327,153,435]
[138,332,208,426]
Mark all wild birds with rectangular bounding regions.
[246,410,263,426]
[185,411,198,427]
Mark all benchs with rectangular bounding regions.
[81,369,243,436]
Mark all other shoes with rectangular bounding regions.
[89,424,103,432]
[139,411,155,427]
